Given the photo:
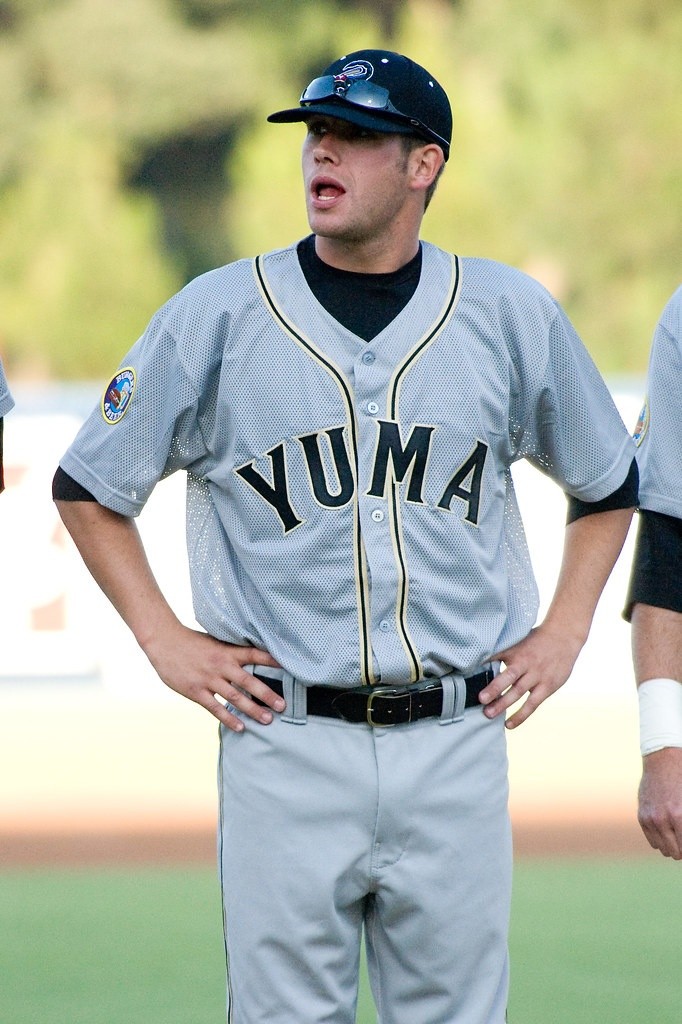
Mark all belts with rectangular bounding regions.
[251,667,495,729]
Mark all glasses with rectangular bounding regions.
[299,76,450,148]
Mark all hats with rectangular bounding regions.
[268,50,453,165]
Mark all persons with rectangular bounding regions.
[52,49,639,1023]
[623,287,682,860]
